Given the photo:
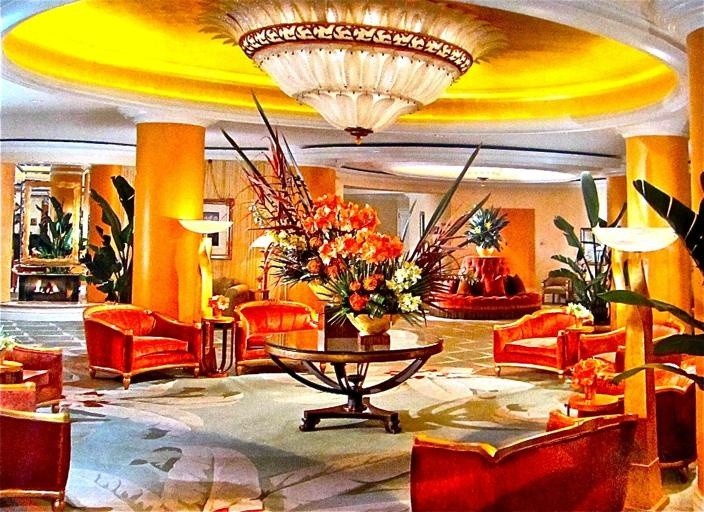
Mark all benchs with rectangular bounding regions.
[429,256,543,321]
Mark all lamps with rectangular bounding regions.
[192,0,513,147]
[249,233,279,301]
[177,217,237,315]
[588,138,680,257]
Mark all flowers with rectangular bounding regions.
[206,294,229,311]
[566,302,589,318]
[218,87,492,328]
[569,358,612,386]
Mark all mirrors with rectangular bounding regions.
[18,163,91,266]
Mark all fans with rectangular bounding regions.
[0,326,17,352]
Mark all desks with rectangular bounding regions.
[260,331,444,435]
[0,360,25,384]
[200,314,236,378]
[568,392,619,419]
[11,263,82,303]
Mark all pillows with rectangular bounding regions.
[443,276,458,295]
[455,279,476,295]
[505,272,526,297]
[483,274,506,297]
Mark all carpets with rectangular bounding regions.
[0,361,580,512]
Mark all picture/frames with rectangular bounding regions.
[203,198,235,261]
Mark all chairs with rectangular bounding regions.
[81,302,202,390]
[408,408,639,512]
[493,308,593,377]
[233,297,319,376]
[211,277,249,321]
[595,379,698,484]
[574,320,685,397]
[541,275,573,304]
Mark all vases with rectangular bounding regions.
[345,309,404,337]
[583,382,598,400]
[0,348,8,364]
[211,307,222,318]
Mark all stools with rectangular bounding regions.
[0,381,72,511]
[0,344,65,412]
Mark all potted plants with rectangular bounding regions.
[465,203,509,258]
